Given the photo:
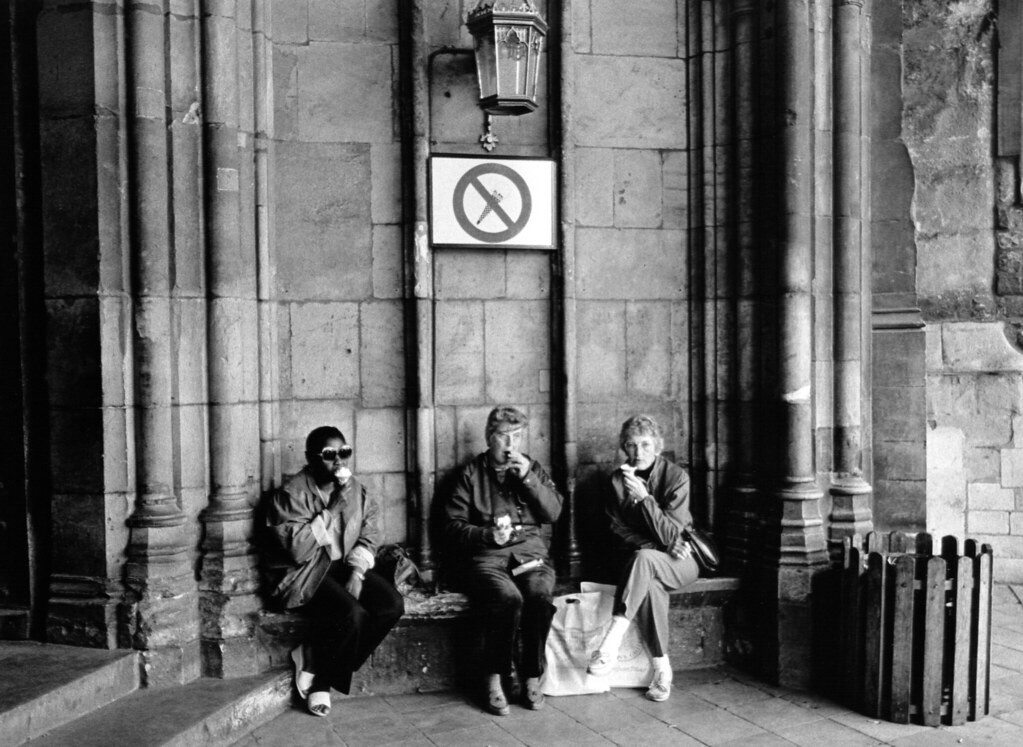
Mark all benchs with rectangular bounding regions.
[259,575,742,699]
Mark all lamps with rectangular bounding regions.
[428,1,551,154]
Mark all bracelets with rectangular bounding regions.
[353,571,364,580]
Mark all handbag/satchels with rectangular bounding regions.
[685,527,720,572]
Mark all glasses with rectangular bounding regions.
[317,444,352,462]
[622,441,656,453]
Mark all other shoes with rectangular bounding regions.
[486,690,511,715]
[585,651,617,677]
[645,668,673,701]
[521,683,544,710]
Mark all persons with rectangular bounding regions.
[266,426,404,718]
[444,406,564,714]
[586,414,700,701]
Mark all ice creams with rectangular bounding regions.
[620,464,637,476]
[335,467,352,487]
[493,513,511,531]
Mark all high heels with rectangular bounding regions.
[307,692,331,717]
[291,645,316,698]
